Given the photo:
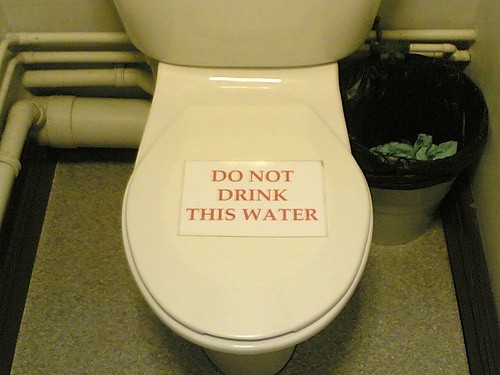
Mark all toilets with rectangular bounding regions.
[113,0,381,375]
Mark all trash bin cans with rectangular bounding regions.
[339,48,489,246]
